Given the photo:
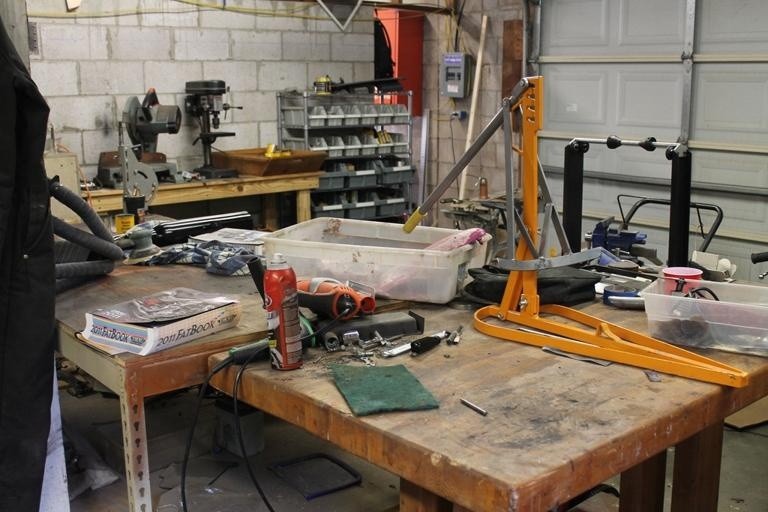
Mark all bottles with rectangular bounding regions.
[262,251,304,370]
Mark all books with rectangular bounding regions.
[186,226,274,257]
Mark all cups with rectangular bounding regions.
[663,266,703,296]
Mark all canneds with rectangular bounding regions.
[316,77,332,94]
[115,214,135,234]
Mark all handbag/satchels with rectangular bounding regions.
[464,261,601,307]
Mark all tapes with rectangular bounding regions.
[604,285,637,306]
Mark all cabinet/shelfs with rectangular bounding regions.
[276,90,414,229]
